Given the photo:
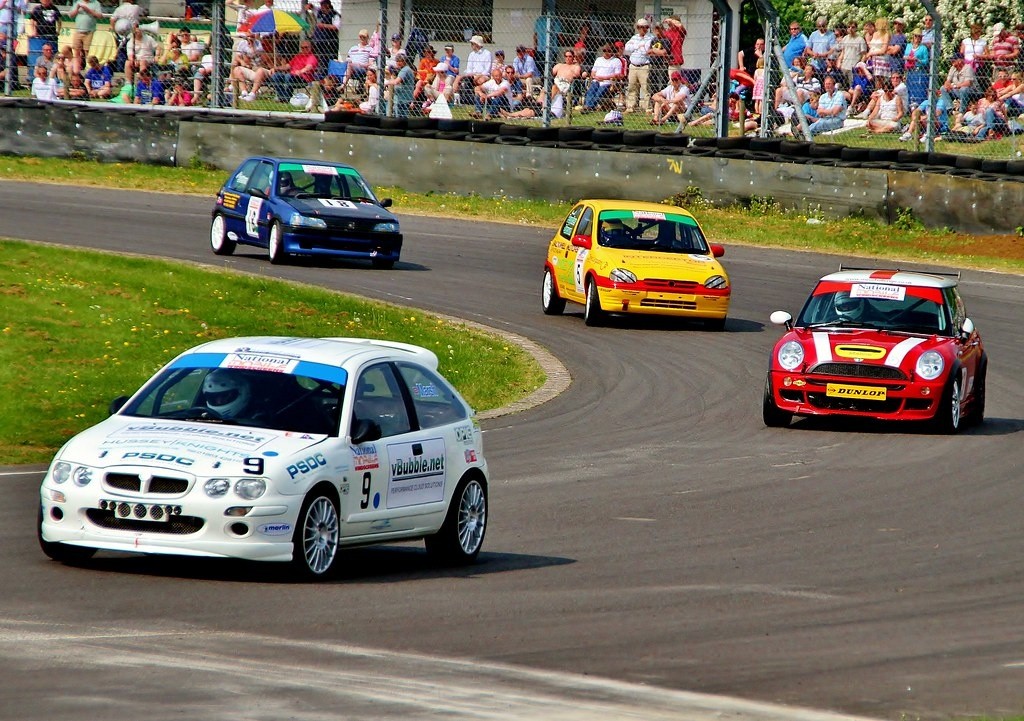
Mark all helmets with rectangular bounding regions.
[201,368,249,418]
[268,169,293,193]
[834,291,864,322]
[603,218,623,242]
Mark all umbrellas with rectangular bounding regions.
[237,9,311,68]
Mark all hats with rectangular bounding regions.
[296,16,1024,94]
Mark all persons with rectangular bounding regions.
[0,0,1024,142]
[264,173,291,195]
[602,220,624,245]
[203,370,252,420]
[832,291,864,322]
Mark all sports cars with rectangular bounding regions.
[539,197,732,329]
[761,263,989,432]
[209,154,407,270]
[34,330,492,583]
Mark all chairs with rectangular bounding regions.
[295,59,347,97]
[586,76,625,109]
[569,72,584,107]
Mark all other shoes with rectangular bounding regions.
[305,100,985,147]
